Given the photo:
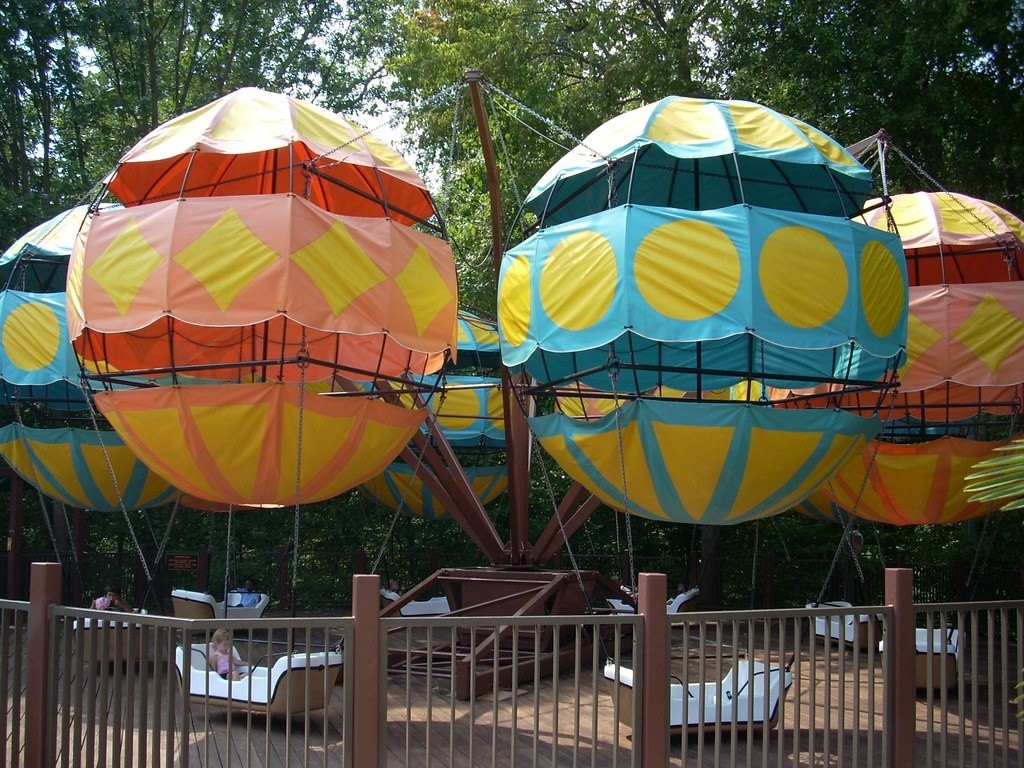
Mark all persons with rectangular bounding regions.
[620,579,638,600]
[209,628,249,680]
[667,583,685,605]
[90,585,133,612]
[389,579,403,597]
